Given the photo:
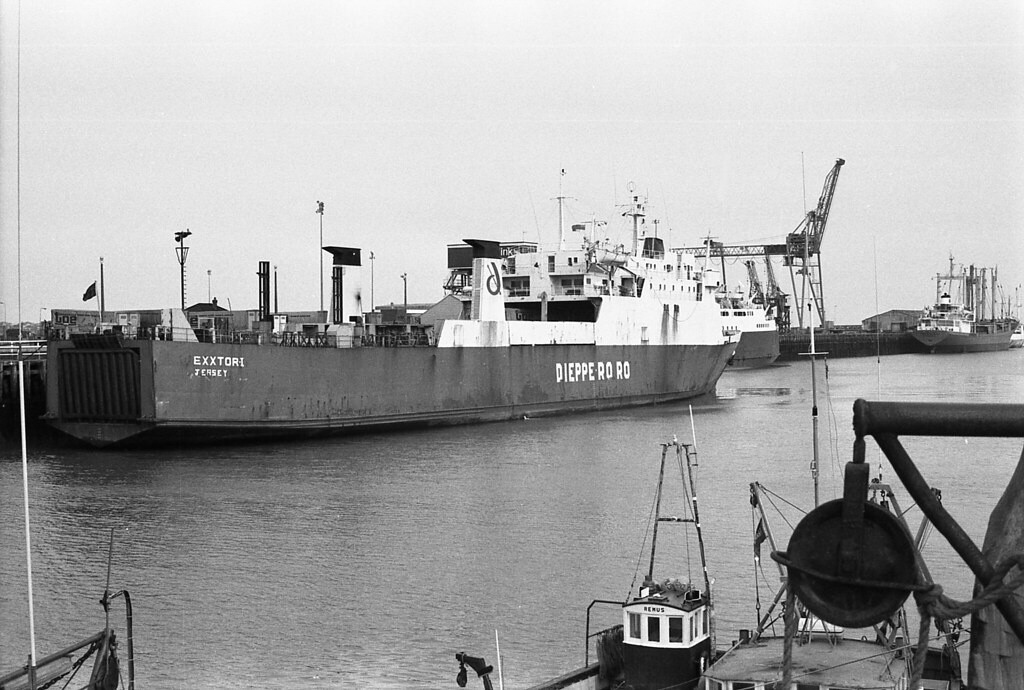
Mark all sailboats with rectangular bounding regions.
[910,251,1024,353]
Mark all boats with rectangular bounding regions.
[570,402,716,690]
[0,360,135,690]
[39,165,743,446]
[694,238,785,369]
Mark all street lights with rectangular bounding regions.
[274,265,279,313]
[401,272,407,313]
[205,269,212,302]
[40,306,46,330]
[314,200,324,312]
[1,302,7,339]
[173,228,192,311]
[368,251,375,311]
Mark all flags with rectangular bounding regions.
[83,283,97,302]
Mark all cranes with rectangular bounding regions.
[669,158,844,334]
